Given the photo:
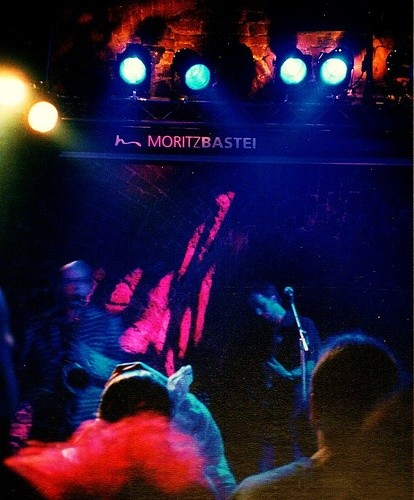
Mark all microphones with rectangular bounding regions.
[284,287,295,297]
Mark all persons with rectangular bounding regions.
[243,282,320,466]
[0,336,414,500]
[28,261,121,437]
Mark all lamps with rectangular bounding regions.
[315,46,354,90]
[380,36,413,95]
[276,48,313,88]
[175,50,212,91]
[117,43,153,86]
[23,90,61,136]
